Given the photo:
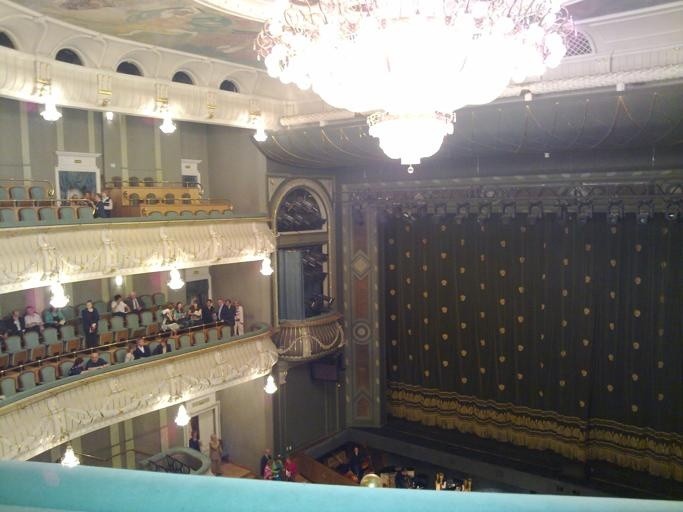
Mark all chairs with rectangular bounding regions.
[0,291,232,397]
[0,176,234,223]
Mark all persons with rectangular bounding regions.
[110,294,130,318]
[262,458,273,480]
[81,299,99,354]
[151,336,171,355]
[65,184,113,219]
[272,451,282,480]
[161,296,244,336]
[208,432,223,475]
[124,290,145,313]
[85,352,110,369]
[349,445,363,475]
[284,454,296,482]
[260,448,271,475]
[131,336,150,359]
[23,305,45,333]
[42,304,66,327]
[66,357,87,375]
[3,308,25,337]
[188,429,200,452]
[123,343,136,363]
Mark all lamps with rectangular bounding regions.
[252,0,577,176]
[60,409,80,467]
